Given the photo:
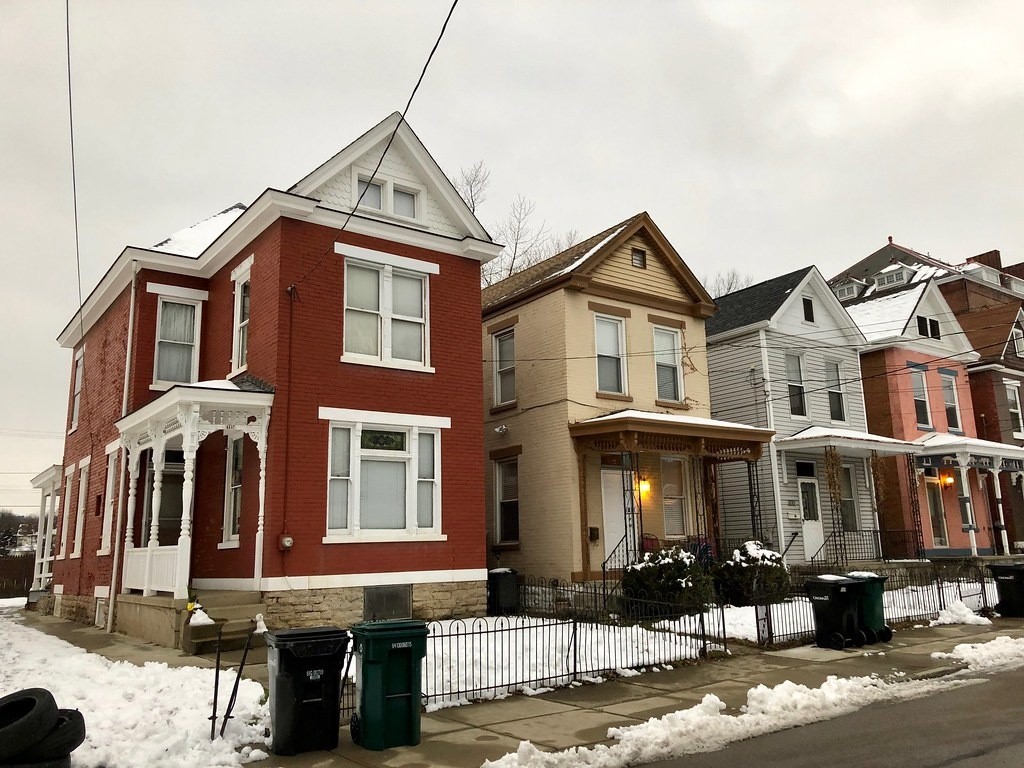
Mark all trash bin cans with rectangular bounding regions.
[349,618,430,752]
[840,571,893,645]
[487,566,519,616]
[261,625,350,757]
[803,573,867,651]
[983,563,1024,618]
